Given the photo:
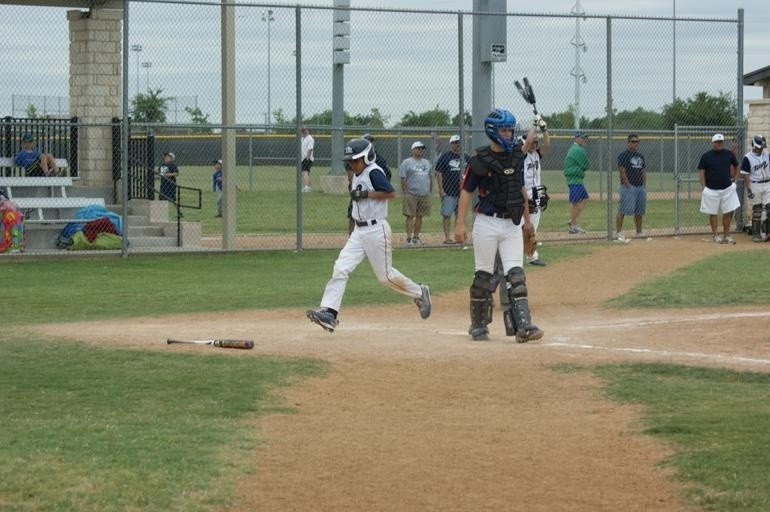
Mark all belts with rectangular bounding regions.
[486,211,510,219]
[752,180,769,183]
[357,220,376,226]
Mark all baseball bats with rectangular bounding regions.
[515,82,548,131]
[524,78,538,126]
[168,338,253,349]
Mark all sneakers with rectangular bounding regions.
[306,309,336,333]
[415,285,432,318]
[530,259,545,266]
[473,333,488,341]
[516,330,543,342]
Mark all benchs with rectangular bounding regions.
[0,157,109,225]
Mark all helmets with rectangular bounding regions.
[751,135,766,150]
[521,134,539,148]
[484,109,519,152]
[340,138,376,165]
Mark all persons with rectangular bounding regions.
[399,141,435,248]
[300,127,314,193]
[14,133,67,178]
[518,117,552,268]
[304,139,431,333]
[211,158,243,219]
[740,135,770,243]
[436,134,469,244]
[344,134,391,234]
[148,151,180,203]
[613,132,649,240]
[562,127,591,235]
[454,109,544,345]
[696,131,740,245]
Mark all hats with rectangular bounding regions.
[411,141,424,150]
[711,134,724,142]
[449,135,461,144]
[574,131,587,137]
[23,135,33,142]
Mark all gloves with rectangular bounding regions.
[539,120,547,132]
[533,113,542,126]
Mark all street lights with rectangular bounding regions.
[142,61,152,98]
[260,9,274,125]
[130,43,140,107]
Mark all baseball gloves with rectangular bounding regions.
[522,223,536,257]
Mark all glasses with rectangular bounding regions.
[452,142,460,144]
[631,141,639,143]
[417,147,424,150]
